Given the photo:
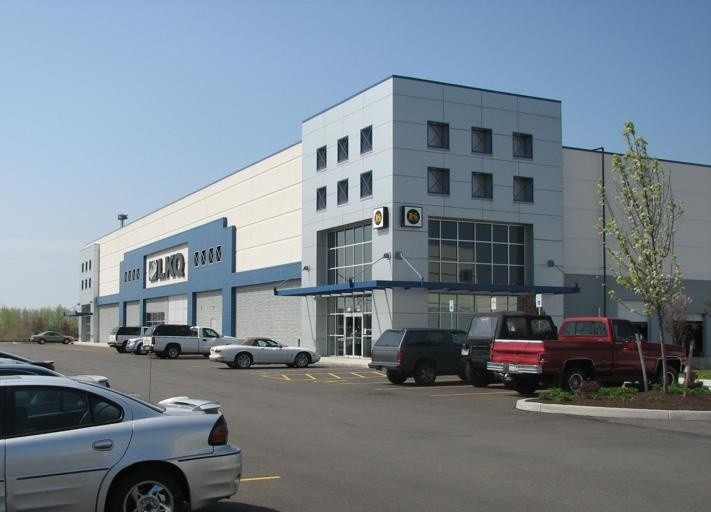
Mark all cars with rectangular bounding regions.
[332,326,374,350]
[30,331,75,344]
[0,351,243,511]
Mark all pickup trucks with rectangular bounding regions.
[485,316,687,394]
[108,325,246,360]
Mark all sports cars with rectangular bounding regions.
[209,336,321,370]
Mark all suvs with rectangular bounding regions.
[459,309,559,391]
[368,327,470,386]
